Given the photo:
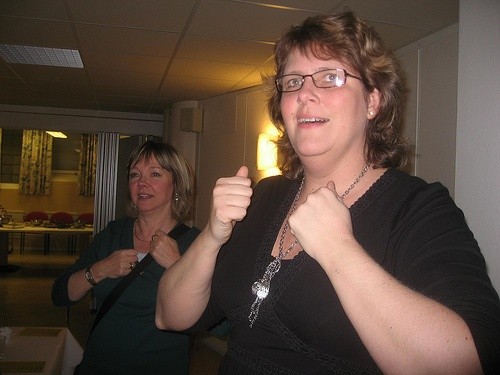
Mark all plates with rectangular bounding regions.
[2,219,94,229]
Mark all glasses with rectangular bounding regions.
[275,69,363,93]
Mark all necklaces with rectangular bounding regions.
[248,159,371,327]
[134,220,151,242]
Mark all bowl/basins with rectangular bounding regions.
[0,217,10,227]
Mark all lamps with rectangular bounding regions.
[256,133,277,170]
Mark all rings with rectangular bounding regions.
[130,263,134,270]
[152,235,157,240]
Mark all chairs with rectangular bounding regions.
[20,211,95,257]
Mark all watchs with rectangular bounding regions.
[85,264,97,286]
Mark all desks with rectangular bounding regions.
[0,221,94,256]
[0,326,85,375]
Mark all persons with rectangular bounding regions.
[156,11,500,375]
[51,139,202,375]
